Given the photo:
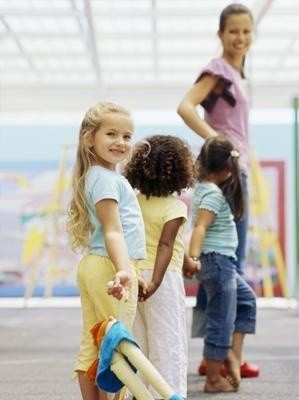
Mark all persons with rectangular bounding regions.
[68,103,147,399]
[185,136,258,394]
[120,132,197,399]
[86,314,184,399]
[177,1,262,379]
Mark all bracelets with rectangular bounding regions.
[190,256,201,262]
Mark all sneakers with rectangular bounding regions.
[198,363,259,377]
